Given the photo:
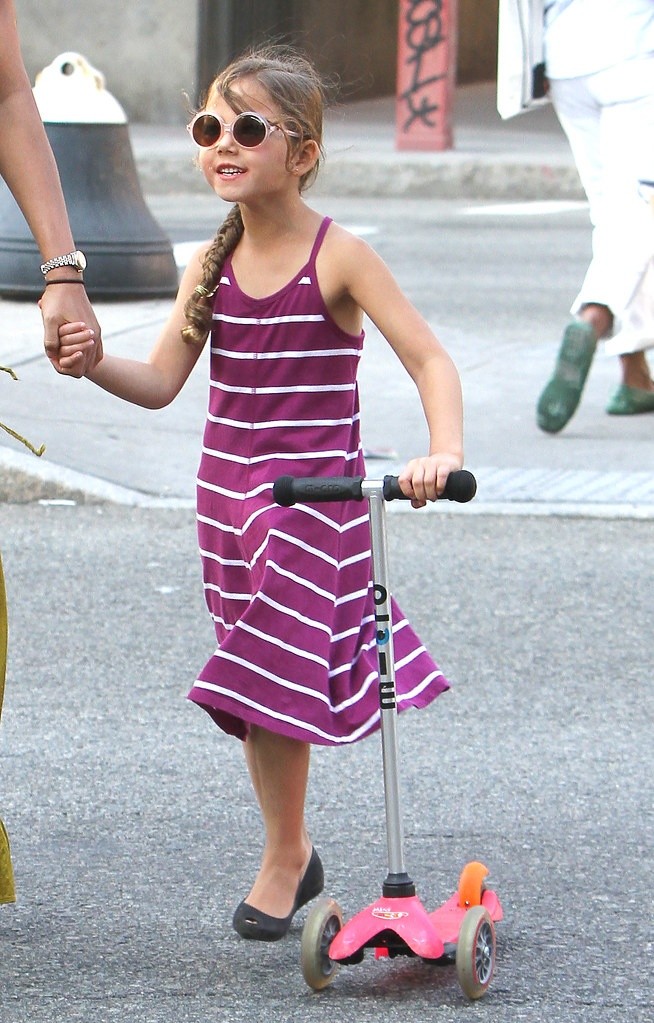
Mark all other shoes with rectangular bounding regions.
[233,844,326,941]
[606,385,654,413]
[536,320,596,433]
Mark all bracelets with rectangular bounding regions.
[45,278,87,285]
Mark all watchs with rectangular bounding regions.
[41,251,87,275]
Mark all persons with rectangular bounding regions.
[38,57,464,942]
[0,0,104,904]
[535,0,654,432]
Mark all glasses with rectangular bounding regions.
[185,110,304,148]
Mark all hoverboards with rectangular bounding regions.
[272,470,505,1002]
